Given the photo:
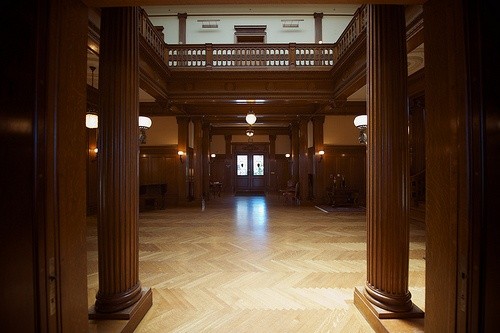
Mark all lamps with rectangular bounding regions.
[139,104,152,130]
[178,151,184,162]
[246,106,257,125]
[353,115,367,144]
[246,127,254,137]
[86,66,99,128]
[319,150,325,159]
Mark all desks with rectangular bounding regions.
[209,182,222,200]
